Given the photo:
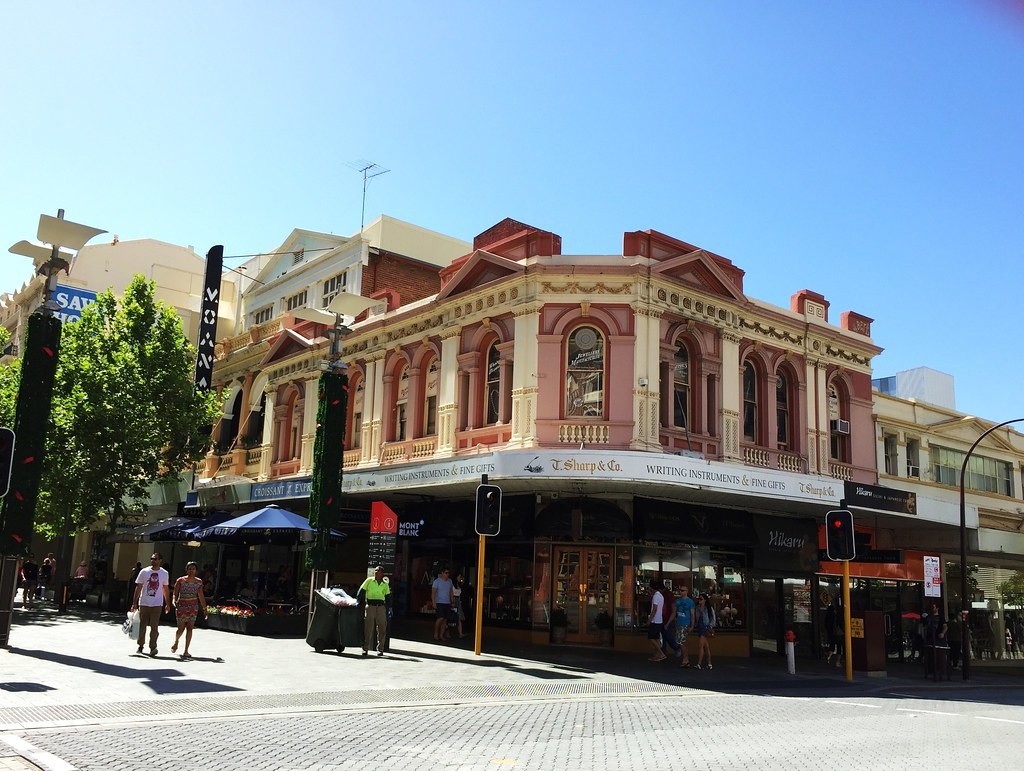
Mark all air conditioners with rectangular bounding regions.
[831,418,851,434]
[908,465,919,477]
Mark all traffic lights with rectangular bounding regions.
[0,426,14,497]
[476,484,501,536]
[826,510,855,562]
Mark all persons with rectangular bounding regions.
[948,606,1024,670]
[664,584,696,668]
[197,559,273,608]
[694,593,717,671]
[431,567,468,642]
[171,562,209,658]
[21,552,57,611]
[355,566,392,657]
[130,552,170,656]
[644,578,682,671]
[824,607,844,668]
[73,560,105,603]
[129,561,143,611]
[921,603,949,682]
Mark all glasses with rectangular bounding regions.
[679,589,687,592]
[444,571,449,574]
[699,598,704,600]
[151,558,159,561]
[29,556,35,558]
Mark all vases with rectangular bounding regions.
[206,614,306,635]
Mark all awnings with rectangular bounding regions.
[114,515,200,543]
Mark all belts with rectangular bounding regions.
[369,603,384,606]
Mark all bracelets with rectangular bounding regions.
[203,607,207,610]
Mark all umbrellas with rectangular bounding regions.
[192,502,350,601]
[167,509,258,598]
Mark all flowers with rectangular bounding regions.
[206,606,304,620]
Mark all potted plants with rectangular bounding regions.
[594,610,614,646]
[550,609,570,644]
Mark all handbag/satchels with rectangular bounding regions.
[122,610,141,640]
[448,609,458,628]
[836,626,844,636]
[706,627,715,637]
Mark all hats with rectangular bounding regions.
[649,580,659,591]
[80,561,87,566]
[185,562,198,569]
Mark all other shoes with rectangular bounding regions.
[362,650,367,656]
[377,650,383,656]
[647,654,712,671]
[434,634,440,641]
[172,644,177,652]
[183,653,191,658]
[439,635,447,642]
[459,633,469,639]
[137,647,143,653]
[837,662,843,667]
[150,649,157,656]
[445,634,452,639]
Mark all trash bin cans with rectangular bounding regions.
[924,645,952,681]
[306,589,357,654]
